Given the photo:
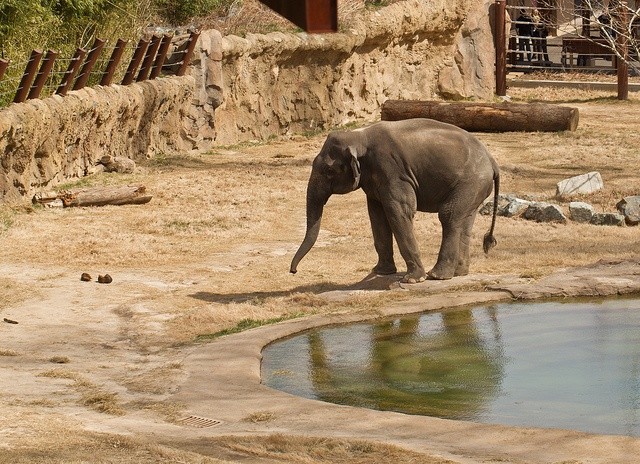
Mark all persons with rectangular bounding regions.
[537,21,550,61]
[598,7,611,38]
[515,9,531,61]
[528,8,541,59]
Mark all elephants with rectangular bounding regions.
[289,117,500,284]
